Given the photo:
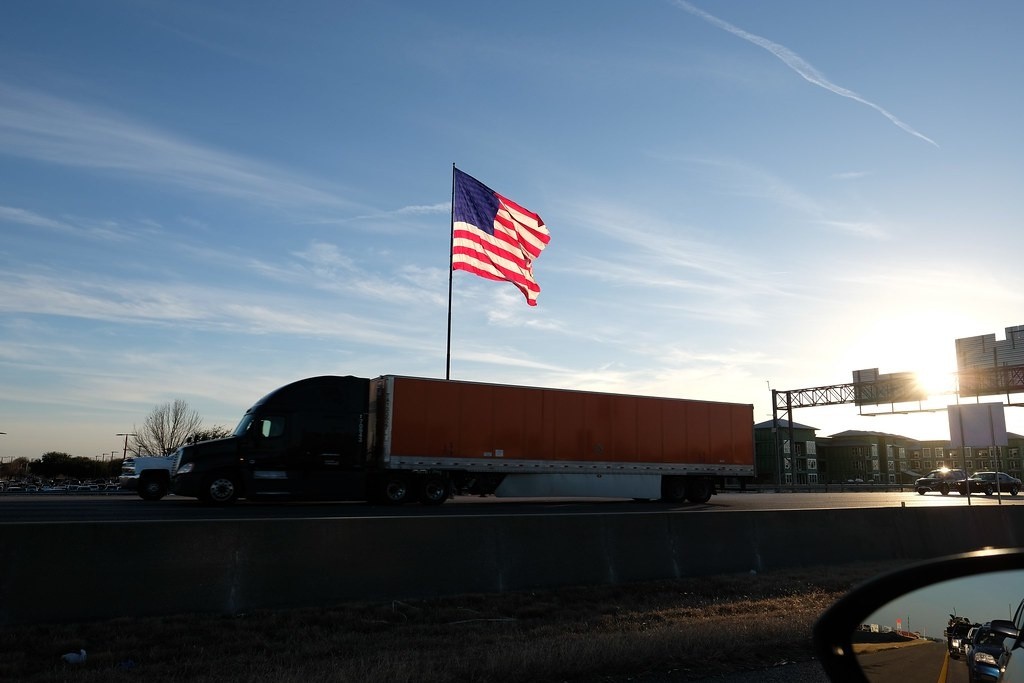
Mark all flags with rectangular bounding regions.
[451,168,550,306]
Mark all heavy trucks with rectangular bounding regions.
[169,375,756,511]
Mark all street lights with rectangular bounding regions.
[117,434,137,459]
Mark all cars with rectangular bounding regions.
[954,471,1022,496]
[914,468,970,495]
[943,614,1005,683]
[119,454,176,502]
[0,479,121,492]
